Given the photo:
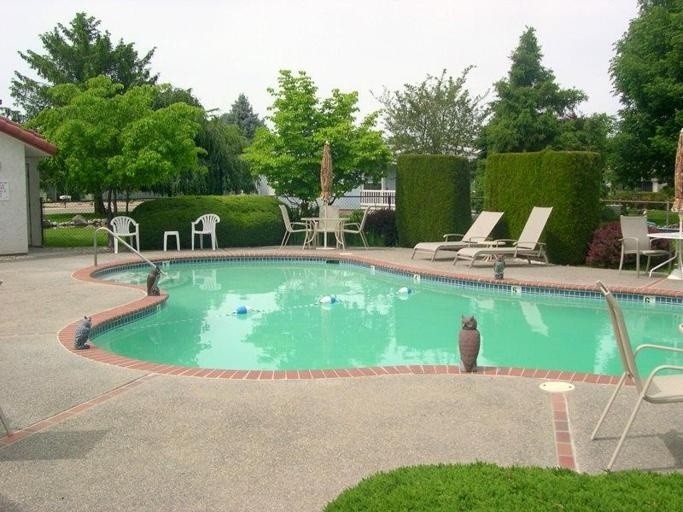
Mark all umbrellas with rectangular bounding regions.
[315,140,336,250]
[667,127,683,280]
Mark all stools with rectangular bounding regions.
[163,230,180,252]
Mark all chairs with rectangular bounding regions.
[616,214,671,279]
[410,210,503,264]
[590,279,681,470]
[452,205,553,267]
[109,216,139,253]
[342,206,370,247]
[190,213,220,250]
[278,204,310,250]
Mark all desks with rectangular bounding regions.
[645,232,682,280]
[300,217,349,250]
